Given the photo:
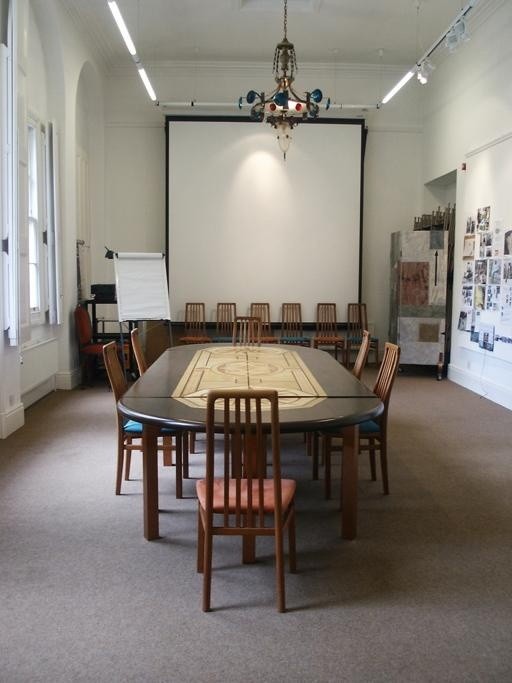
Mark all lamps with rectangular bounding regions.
[238,1,332,160]
[375,45,415,110]
[415,1,479,87]
[105,0,166,106]
